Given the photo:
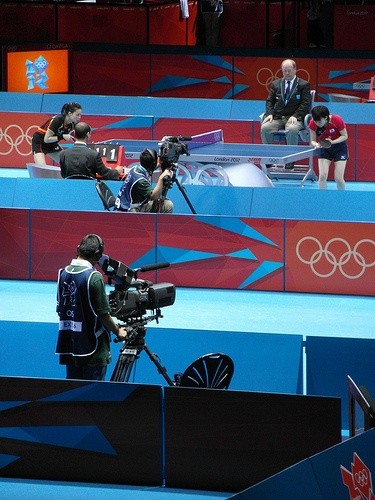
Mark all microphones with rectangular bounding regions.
[134,262,170,272]
[177,135,193,141]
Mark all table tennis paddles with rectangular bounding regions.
[315,139,331,148]
[64,130,76,142]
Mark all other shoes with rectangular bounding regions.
[265,164,272,168]
[284,162,294,172]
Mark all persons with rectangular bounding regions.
[113,148,175,213]
[308,105,349,189]
[59,122,125,181]
[53,234,130,380]
[259,59,311,170]
[31,102,82,166]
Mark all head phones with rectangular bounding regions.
[77,233,104,260]
[146,148,157,168]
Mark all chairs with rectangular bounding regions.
[177,353,236,388]
[96,181,117,211]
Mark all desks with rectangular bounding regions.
[93,129,317,178]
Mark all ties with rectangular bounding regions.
[284,81,290,102]
